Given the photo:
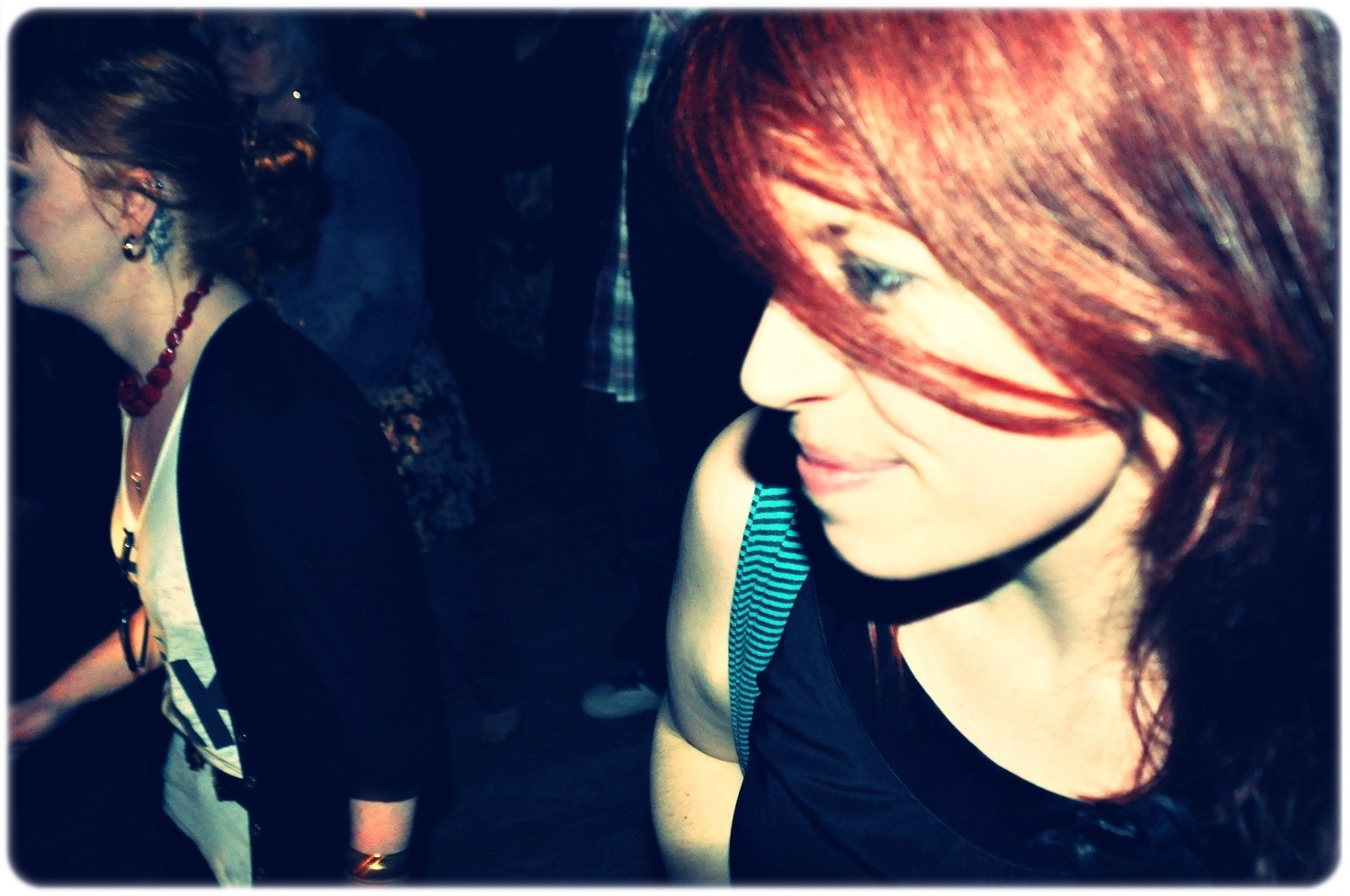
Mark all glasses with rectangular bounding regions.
[110,527,150,672]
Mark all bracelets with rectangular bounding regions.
[343,847,415,883]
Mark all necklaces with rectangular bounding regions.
[114,268,215,418]
[130,422,153,496]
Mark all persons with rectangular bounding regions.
[650,10,1340,887]
[557,11,704,720]
[199,13,479,530]
[10,47,442,885]
